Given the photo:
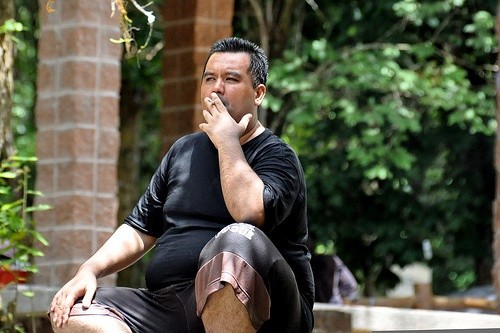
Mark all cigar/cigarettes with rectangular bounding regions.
[207,99,214,105]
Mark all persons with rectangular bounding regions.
[47,36,316,333]
